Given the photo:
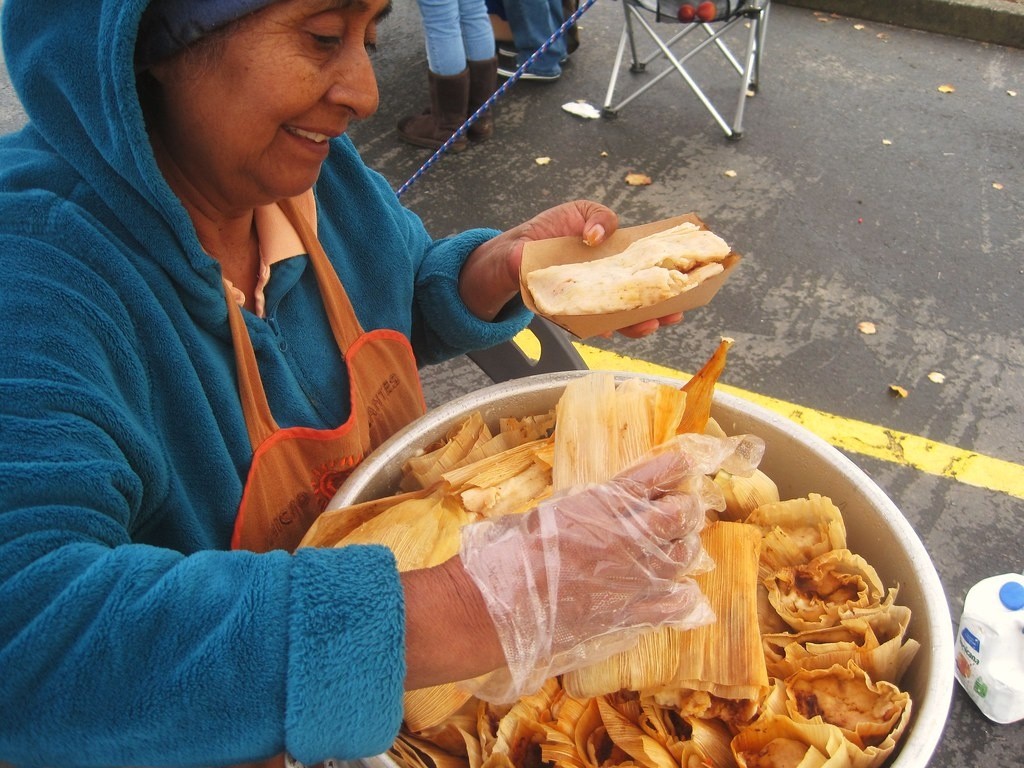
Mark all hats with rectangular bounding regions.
[133,0,279,70]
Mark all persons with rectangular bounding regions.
[496,0,569,78]
[3,2,706,768]
[396,0,499,152]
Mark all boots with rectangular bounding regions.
[395,63,470,153]
[421,55,502,144]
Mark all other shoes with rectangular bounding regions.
[498,41,567,62]
[497,55,561,80]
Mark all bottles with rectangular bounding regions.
[953,573,1024,724]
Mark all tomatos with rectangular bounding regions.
[677,1,716,22]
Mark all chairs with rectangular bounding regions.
[602,1,772,140]
